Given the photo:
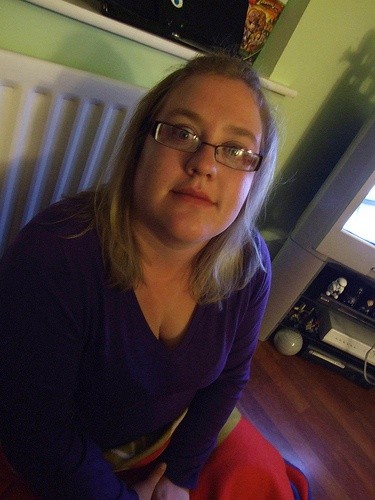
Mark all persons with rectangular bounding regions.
[0,53,279,499]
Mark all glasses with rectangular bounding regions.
[148,120,264,172]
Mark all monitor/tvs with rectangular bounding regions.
[291,110,375,281]
[101,0,249,57]
